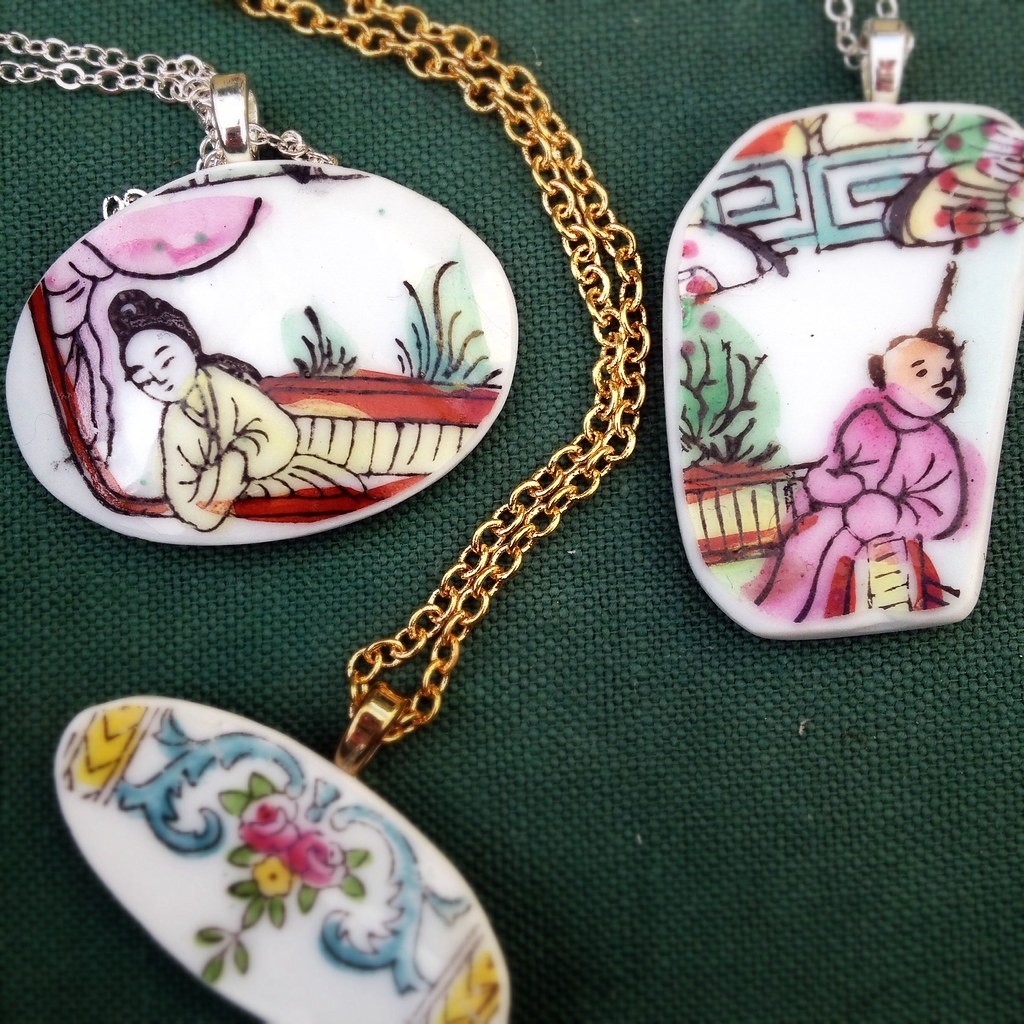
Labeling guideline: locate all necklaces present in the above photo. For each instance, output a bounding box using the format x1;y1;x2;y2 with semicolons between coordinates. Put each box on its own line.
1;29;519;547
53;1;650;1024
661;0;1024;641
